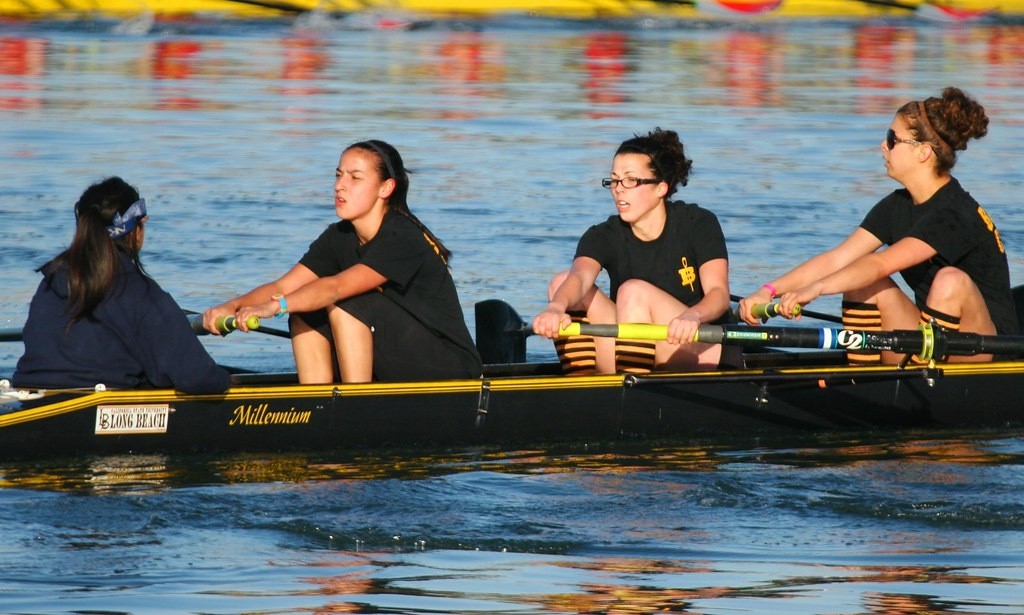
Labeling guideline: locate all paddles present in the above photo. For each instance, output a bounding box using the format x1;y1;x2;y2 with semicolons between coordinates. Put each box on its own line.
928;1;1002;19
557;319;1024;356
0;311;259;343
473;298;802;364
228;0;412;29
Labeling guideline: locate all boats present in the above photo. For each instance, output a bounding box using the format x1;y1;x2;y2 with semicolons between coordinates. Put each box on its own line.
0;288;1024;469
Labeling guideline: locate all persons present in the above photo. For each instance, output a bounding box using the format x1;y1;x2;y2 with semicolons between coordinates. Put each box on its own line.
738;86;1019;366
203;139;484;384
532;127;743;375
8;177;231;396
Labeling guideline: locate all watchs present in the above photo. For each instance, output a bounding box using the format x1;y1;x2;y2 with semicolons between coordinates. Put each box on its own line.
271;293;287;318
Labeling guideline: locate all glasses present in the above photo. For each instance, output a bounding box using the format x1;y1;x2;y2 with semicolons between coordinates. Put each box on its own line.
602;177;662;188
887;128;940;158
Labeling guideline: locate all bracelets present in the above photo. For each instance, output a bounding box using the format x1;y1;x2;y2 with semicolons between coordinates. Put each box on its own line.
763;283;776;298
